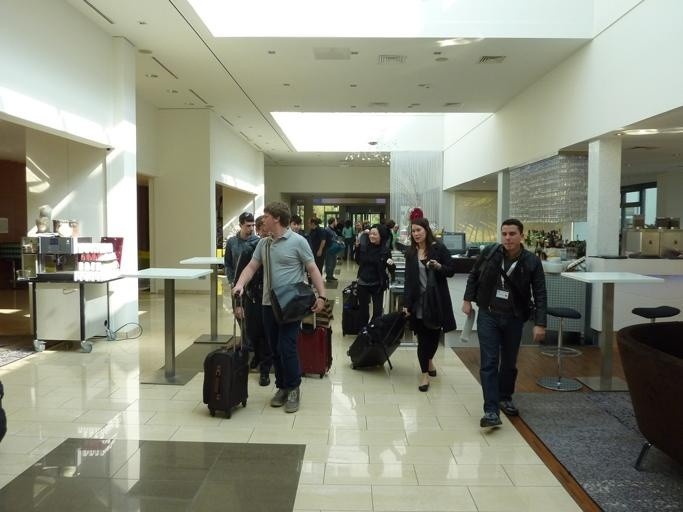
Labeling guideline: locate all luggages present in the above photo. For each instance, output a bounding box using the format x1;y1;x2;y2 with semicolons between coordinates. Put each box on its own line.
298;312;332;378
342;297;370;336
347;311;406;370
202;292;248;419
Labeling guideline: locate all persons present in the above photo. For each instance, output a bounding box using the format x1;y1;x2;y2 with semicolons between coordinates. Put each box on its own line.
463;219;547;427
224;200;457;414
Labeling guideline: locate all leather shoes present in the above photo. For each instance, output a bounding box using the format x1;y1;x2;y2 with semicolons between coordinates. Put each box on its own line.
428;371;436;376
419;384;428;392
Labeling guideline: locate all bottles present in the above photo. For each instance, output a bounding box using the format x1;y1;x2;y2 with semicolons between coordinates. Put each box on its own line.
525;227;565;260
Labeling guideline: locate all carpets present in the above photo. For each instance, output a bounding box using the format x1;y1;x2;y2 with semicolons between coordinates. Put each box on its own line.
511;391;682;512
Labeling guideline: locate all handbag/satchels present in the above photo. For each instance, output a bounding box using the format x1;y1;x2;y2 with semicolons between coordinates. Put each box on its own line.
302;299;334;328
268;282;317;326
342;281;371;306
358;312;405;343
423;268;444;330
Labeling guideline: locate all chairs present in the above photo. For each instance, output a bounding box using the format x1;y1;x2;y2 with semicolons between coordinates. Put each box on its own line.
616;321;681;469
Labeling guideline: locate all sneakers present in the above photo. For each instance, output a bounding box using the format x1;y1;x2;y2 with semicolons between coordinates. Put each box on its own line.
480;412;502;429
271;388;287;406
500;401;518;417
285;387;300;413
260;368;270;386
250;355;259;369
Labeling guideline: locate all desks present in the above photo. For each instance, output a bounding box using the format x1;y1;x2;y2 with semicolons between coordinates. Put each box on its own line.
120;267;214;385
561;271;665;391
179;257;233;343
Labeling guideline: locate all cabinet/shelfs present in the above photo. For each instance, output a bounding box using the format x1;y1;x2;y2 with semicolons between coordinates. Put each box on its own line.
26;273;115;354
641;229;658;259
659;229;682;259
623;229;641;254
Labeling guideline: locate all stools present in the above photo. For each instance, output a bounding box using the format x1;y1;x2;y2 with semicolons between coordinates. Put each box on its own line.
632;306;680;324
535;306;583;393
